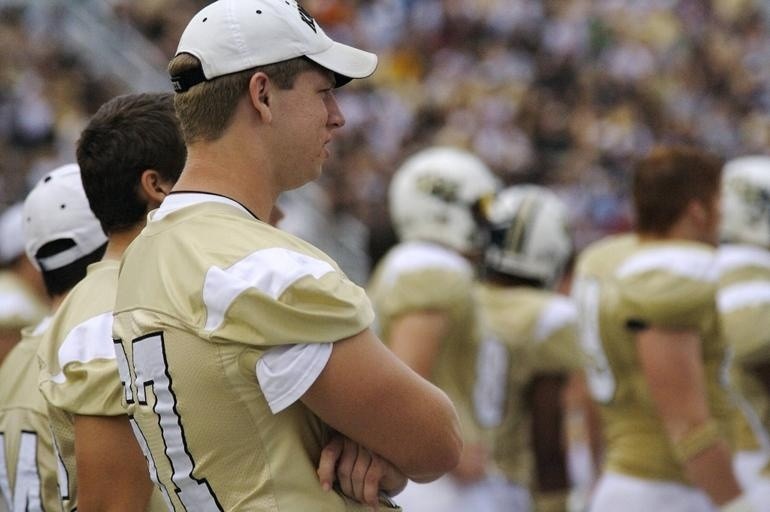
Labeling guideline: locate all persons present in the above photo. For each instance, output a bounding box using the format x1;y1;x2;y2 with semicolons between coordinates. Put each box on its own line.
0;2;767;510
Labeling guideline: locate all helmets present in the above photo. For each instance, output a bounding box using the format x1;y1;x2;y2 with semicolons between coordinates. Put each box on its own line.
387;144;504;259
485;181;576;285
715;152;770;249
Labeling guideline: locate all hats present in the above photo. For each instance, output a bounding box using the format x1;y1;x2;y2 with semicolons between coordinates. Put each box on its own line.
168;0;379;95
0;203;27;266
20;163;108;273
